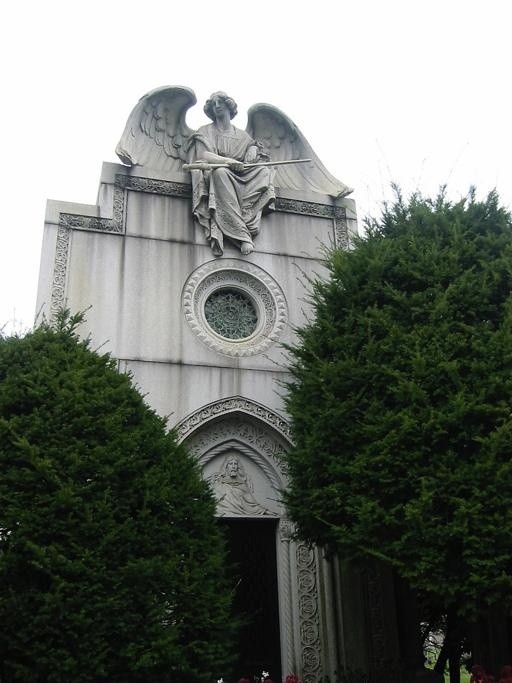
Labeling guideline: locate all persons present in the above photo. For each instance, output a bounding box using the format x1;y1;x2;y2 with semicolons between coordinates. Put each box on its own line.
190;90;276;257
202;455;278;518
